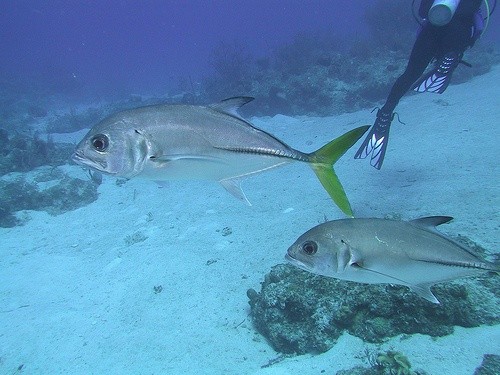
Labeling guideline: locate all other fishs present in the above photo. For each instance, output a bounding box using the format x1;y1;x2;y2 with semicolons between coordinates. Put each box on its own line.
70;96;372;219
285;215;500;304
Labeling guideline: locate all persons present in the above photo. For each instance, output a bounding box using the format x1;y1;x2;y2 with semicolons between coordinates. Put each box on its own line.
353;0;483;170
